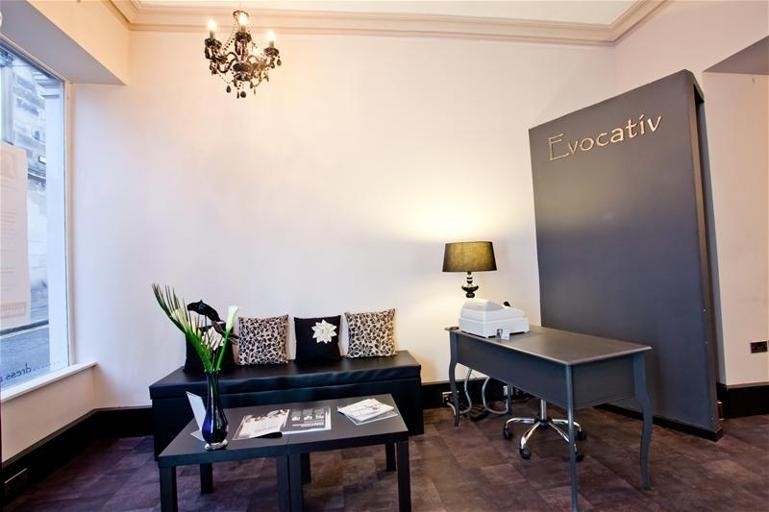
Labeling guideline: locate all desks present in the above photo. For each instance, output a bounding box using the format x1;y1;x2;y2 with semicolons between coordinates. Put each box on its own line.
448;323;652;512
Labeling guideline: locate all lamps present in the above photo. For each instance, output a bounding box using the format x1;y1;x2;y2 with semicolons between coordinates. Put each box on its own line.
442;241;497;298
202;9;281;99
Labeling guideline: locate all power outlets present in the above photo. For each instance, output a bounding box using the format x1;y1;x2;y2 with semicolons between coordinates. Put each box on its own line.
442;391;460;405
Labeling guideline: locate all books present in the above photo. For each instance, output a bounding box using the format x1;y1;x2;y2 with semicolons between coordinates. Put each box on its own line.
232;406;332;441
344;398;398;425
337;398;394;421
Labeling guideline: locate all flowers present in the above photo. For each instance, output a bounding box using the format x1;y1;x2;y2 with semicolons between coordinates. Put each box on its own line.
151;284;240;434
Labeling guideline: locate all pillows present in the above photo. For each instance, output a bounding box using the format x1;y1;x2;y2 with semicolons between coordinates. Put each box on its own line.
345;308;400;358
237;315;289;365
183;322;237;376
293;315;343;365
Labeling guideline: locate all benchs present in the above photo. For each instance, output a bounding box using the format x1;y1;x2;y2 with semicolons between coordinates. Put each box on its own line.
150;351;424;468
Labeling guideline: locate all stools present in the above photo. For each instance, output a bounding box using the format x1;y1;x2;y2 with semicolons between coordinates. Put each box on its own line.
504;398;587;463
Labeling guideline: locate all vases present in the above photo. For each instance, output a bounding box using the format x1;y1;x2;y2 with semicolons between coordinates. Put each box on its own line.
202;370;229;450
